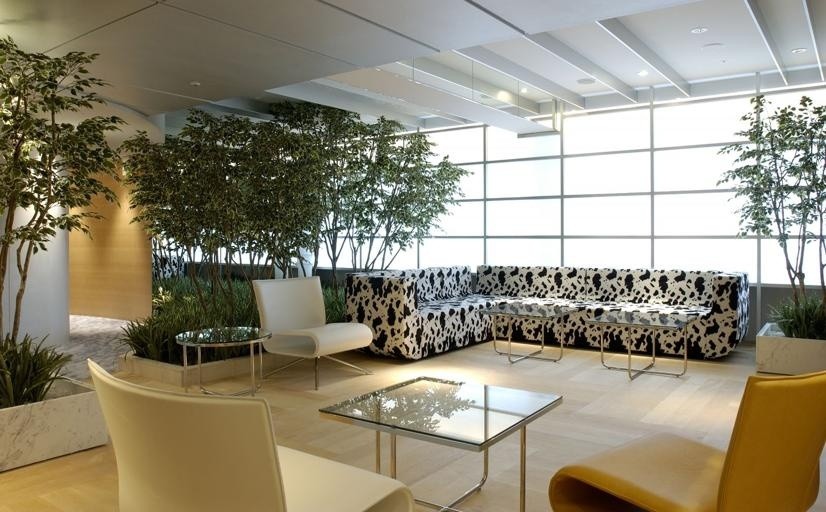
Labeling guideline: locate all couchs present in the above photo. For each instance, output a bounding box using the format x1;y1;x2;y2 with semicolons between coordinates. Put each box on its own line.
346;266;747;361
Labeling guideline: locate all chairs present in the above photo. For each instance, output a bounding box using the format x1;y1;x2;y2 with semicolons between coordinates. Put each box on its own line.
547;370;825;509
86;358;414;512
251;276;374;387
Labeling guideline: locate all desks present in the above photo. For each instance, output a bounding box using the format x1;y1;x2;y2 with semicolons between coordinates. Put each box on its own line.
478;298;580;365
176;326;272;395
583;310;698;380
316;377;562;512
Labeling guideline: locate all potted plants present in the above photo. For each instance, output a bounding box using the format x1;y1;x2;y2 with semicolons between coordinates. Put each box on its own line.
717;88;826;376
0;33;129;474
